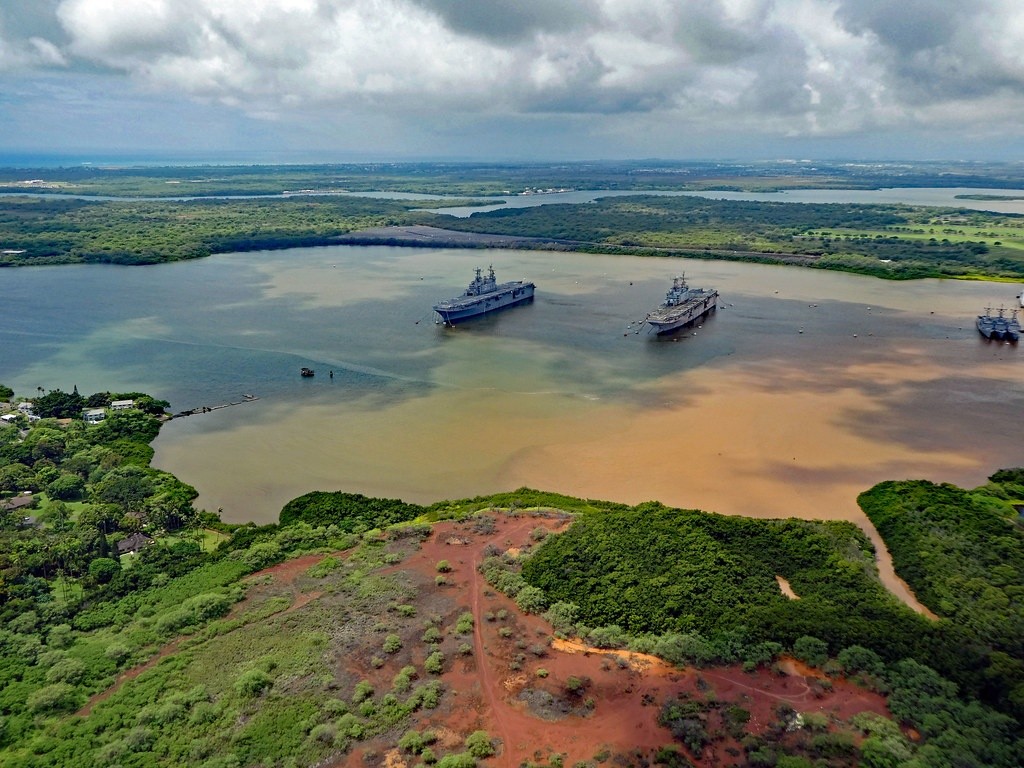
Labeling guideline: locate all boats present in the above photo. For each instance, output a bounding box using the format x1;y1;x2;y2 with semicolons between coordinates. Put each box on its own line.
301;367;315;377
645;272;719;335
432;264;536;325
994;304;1008;338
977;303;994;337
1016;291;1024;308
1009;306;1022;340
